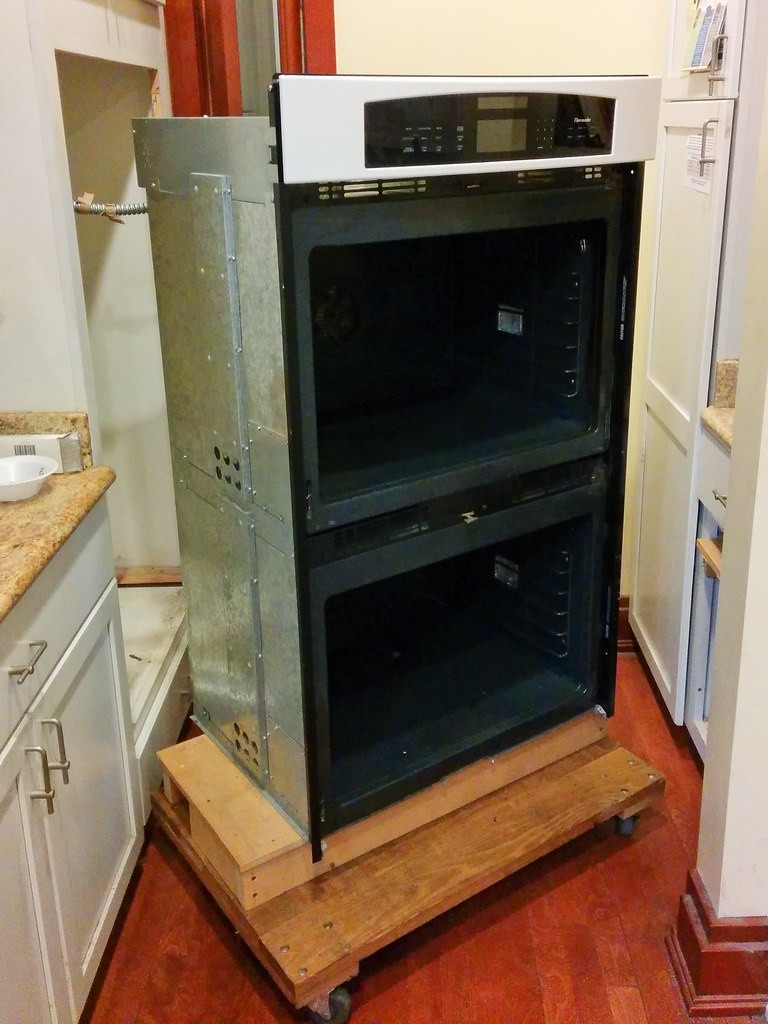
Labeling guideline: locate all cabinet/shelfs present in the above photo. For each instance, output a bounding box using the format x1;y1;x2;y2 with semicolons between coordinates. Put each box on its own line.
0;495;146;1024
627;0;758;727
684;424;731;762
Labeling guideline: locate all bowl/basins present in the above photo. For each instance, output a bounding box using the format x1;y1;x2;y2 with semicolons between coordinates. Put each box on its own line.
0;455;59;502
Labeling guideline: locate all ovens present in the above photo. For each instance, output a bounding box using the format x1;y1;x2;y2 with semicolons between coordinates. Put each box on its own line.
125;72;667;1024
